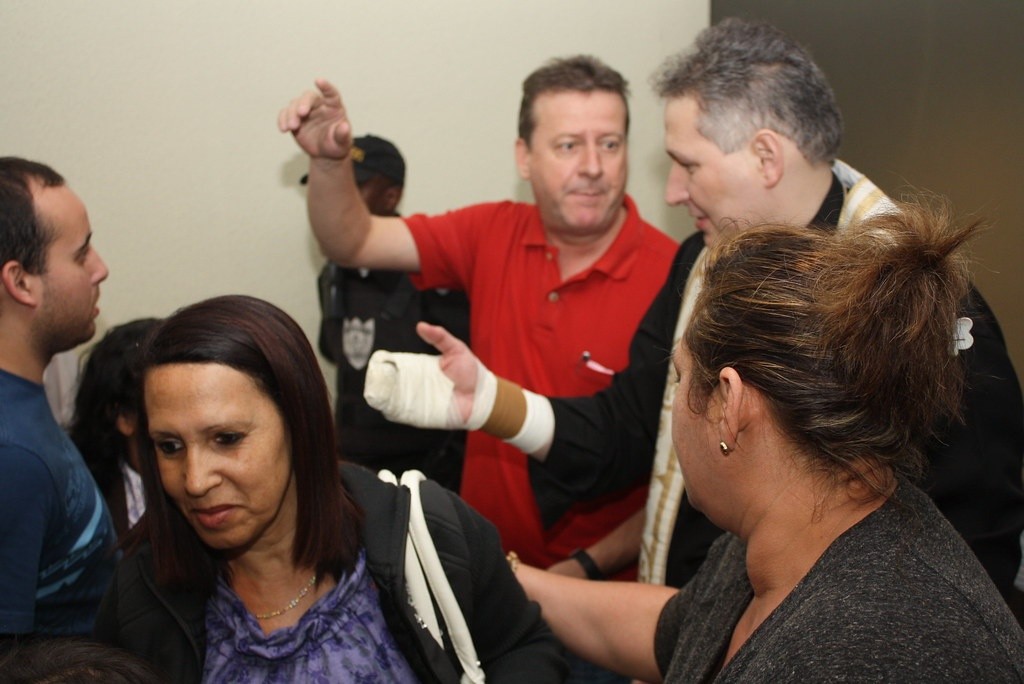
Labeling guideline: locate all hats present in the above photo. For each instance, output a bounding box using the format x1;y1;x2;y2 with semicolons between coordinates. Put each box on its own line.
300;135;405;187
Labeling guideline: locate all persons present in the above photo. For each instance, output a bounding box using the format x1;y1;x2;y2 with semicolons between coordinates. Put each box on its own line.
69;315;168;540
0;155;124;641
502;205;1024;684
0;633;163;684
137;293;566;684
362;16;1024;628
298;135;470;495
276;54;682;582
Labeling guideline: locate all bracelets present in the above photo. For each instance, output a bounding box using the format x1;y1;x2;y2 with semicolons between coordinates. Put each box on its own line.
570;548;605;581
505;551;520;573
